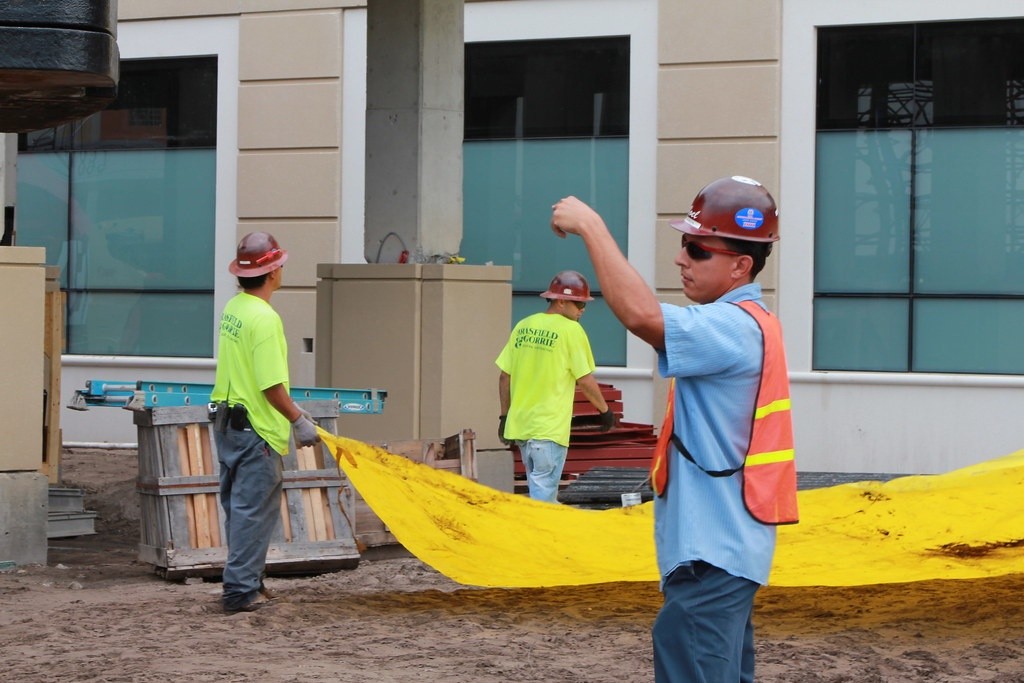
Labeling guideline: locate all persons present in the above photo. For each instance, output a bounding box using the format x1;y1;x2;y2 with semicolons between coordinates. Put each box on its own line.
497;270;614;507
549;176;797;683
207;232;321;613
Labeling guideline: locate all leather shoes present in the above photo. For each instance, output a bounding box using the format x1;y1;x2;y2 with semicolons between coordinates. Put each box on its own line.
223;588;281;614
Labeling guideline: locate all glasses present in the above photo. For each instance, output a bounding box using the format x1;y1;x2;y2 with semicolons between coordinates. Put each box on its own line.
681;233;743;260
571;300;586;310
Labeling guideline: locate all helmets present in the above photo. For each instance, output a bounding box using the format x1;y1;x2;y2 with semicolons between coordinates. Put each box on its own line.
229;231;289;278
669;174;781;243
540;270;595;302
